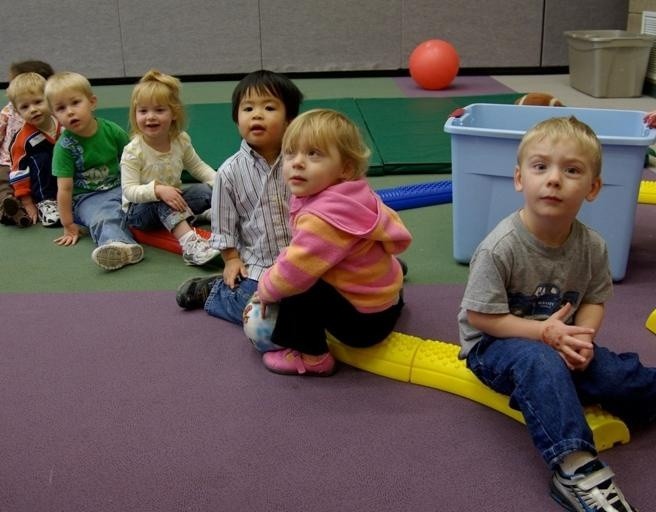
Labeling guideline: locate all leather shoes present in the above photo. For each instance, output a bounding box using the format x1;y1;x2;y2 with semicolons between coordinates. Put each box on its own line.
261;347;336;377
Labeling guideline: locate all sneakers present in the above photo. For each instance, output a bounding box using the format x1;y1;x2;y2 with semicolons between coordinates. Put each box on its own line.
91;241;144;271
548;457;639;512
182;230;221;265
176;274;223;308
2;194;64;228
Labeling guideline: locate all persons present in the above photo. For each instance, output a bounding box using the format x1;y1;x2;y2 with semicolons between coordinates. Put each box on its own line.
4;71;62;227
119;70;222;265
176;69;306;325
0;60;56;227
253;109;414;377
456;116;656;512
43;71;144;270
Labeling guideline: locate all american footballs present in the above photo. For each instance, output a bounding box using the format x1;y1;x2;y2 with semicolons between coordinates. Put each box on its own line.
513;91;565;107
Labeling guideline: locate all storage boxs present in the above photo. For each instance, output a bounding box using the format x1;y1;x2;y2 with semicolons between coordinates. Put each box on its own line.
627;14;656;84
628;0;656;14
564;29;653;98
443;103;656;283
